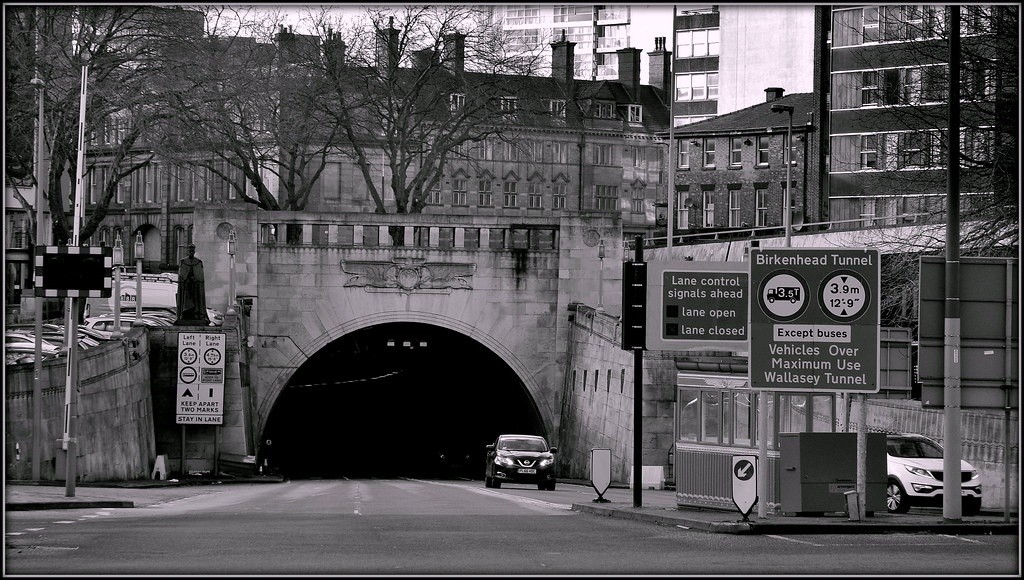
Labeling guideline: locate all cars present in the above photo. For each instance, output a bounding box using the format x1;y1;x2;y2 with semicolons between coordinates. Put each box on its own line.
485;433;558;492
883;433;982;517
5;270;225;359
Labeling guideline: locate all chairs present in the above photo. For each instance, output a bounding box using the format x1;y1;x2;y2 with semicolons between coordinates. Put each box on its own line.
899;444;916;455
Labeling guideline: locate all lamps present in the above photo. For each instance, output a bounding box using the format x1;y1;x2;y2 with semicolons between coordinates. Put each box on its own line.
691;141;700;147
743;138;752;146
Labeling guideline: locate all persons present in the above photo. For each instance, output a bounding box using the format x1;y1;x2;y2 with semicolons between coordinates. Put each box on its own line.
172;244;210;327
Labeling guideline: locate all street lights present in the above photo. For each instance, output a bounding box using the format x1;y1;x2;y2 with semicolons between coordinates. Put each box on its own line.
770;103;795;248
28;76;42;487
